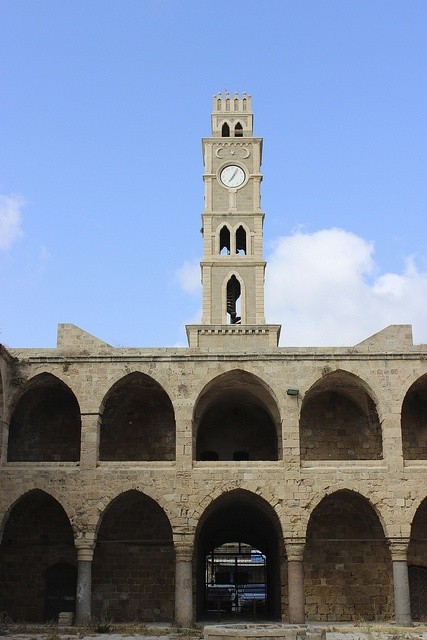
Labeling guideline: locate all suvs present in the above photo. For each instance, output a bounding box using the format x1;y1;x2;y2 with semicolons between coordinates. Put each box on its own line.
206;585;233;612
238;584;267;612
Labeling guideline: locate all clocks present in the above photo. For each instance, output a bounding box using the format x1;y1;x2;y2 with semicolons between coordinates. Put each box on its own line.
220;165;245;189
